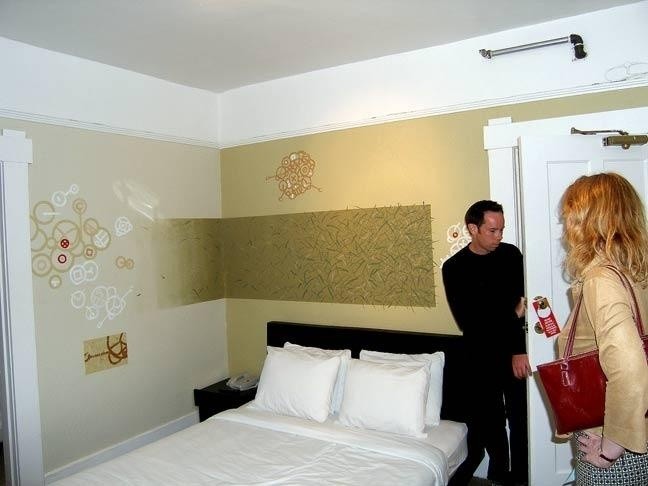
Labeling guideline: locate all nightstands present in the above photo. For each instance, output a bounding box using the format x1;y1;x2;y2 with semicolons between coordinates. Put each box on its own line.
194;378;258;423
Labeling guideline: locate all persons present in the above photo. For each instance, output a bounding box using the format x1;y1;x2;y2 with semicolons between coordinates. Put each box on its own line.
441;201;532;486
536;172;648;486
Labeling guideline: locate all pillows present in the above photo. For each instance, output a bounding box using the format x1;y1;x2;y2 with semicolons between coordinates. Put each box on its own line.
341;356;431;439
283;342;351;422
359;350;445;426
255;345;341;422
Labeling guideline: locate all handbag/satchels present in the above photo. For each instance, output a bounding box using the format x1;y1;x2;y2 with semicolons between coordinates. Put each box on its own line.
535;265;648;432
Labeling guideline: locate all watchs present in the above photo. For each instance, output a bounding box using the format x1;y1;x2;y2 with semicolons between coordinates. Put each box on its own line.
597;447;621;466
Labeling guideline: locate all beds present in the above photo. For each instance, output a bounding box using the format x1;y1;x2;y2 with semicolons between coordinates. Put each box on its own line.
47;321;486;486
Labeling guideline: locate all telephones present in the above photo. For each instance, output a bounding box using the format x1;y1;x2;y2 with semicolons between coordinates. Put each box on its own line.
225;372;260;391
